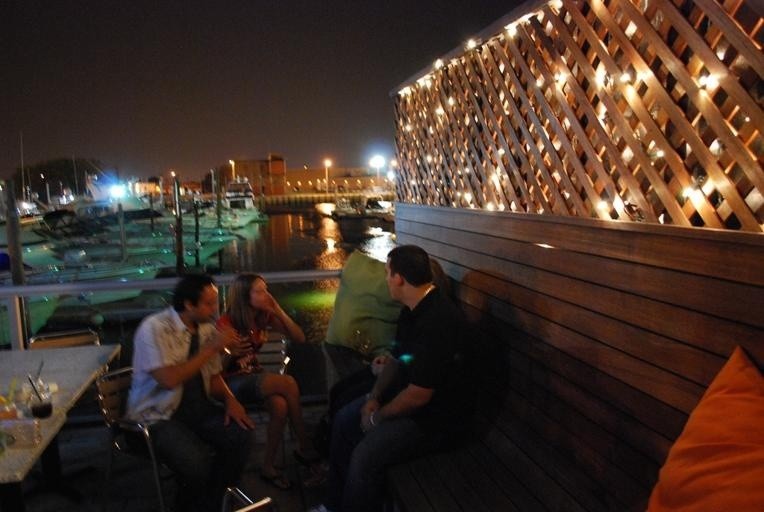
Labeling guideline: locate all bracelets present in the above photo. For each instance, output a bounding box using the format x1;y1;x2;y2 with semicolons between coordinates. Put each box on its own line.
370;411;375;427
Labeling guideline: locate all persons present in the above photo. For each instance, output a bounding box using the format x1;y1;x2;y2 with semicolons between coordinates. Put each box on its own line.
215;272;324;493
311;243;465;512
327;257;447;412
120;271;254;511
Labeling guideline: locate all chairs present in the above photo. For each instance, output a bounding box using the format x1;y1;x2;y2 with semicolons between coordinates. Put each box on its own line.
95;330;290;510
31;331;103;416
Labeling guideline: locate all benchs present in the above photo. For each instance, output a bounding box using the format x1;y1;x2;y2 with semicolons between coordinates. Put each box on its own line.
320;202;763;510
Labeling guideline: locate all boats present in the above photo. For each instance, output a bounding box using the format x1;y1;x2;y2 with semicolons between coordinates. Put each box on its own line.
332;199;395;250
0;197;268;345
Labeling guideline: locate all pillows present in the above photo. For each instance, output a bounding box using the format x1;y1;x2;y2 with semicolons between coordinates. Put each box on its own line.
644;342;764;511
324;248;399;354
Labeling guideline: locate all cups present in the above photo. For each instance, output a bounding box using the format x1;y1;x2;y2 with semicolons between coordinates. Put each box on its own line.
29;392;53;418
213;316;232;334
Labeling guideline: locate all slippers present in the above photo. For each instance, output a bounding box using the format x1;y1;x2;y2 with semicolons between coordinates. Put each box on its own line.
259;470;295;492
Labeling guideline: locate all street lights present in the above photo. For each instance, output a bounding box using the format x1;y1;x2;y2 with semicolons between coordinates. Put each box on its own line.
370;156;383;192
229;159;237;185
323;159;332;192
